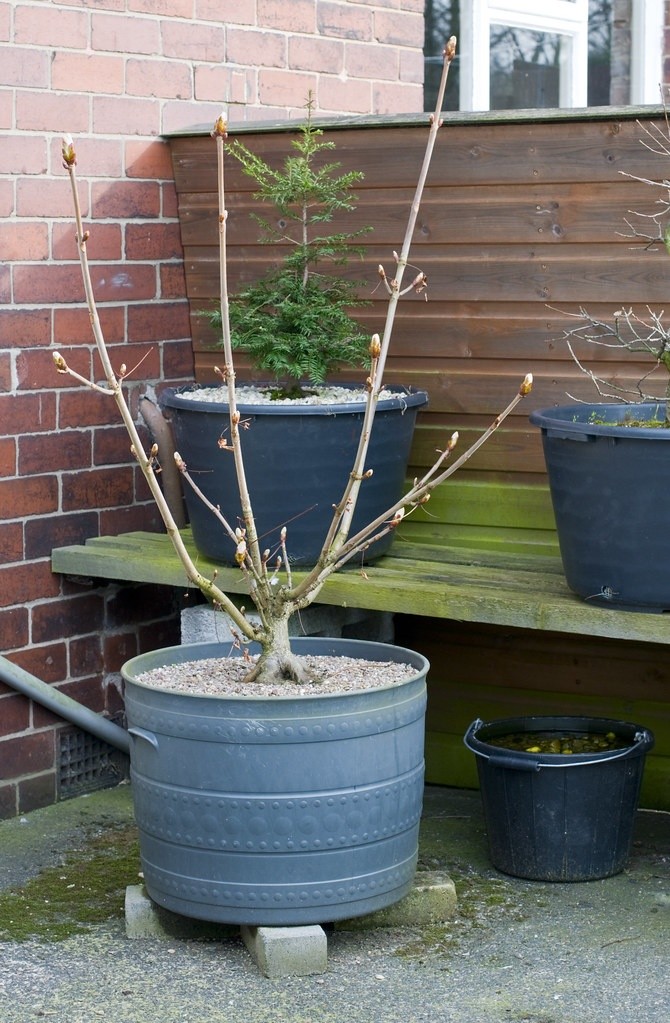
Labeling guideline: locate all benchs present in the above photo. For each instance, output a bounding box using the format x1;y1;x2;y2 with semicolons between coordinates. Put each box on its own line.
50;102;668;650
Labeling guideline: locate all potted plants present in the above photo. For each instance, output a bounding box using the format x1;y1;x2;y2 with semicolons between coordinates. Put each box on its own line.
161;86;432;571
62;33;534;927
531;80;670;613
